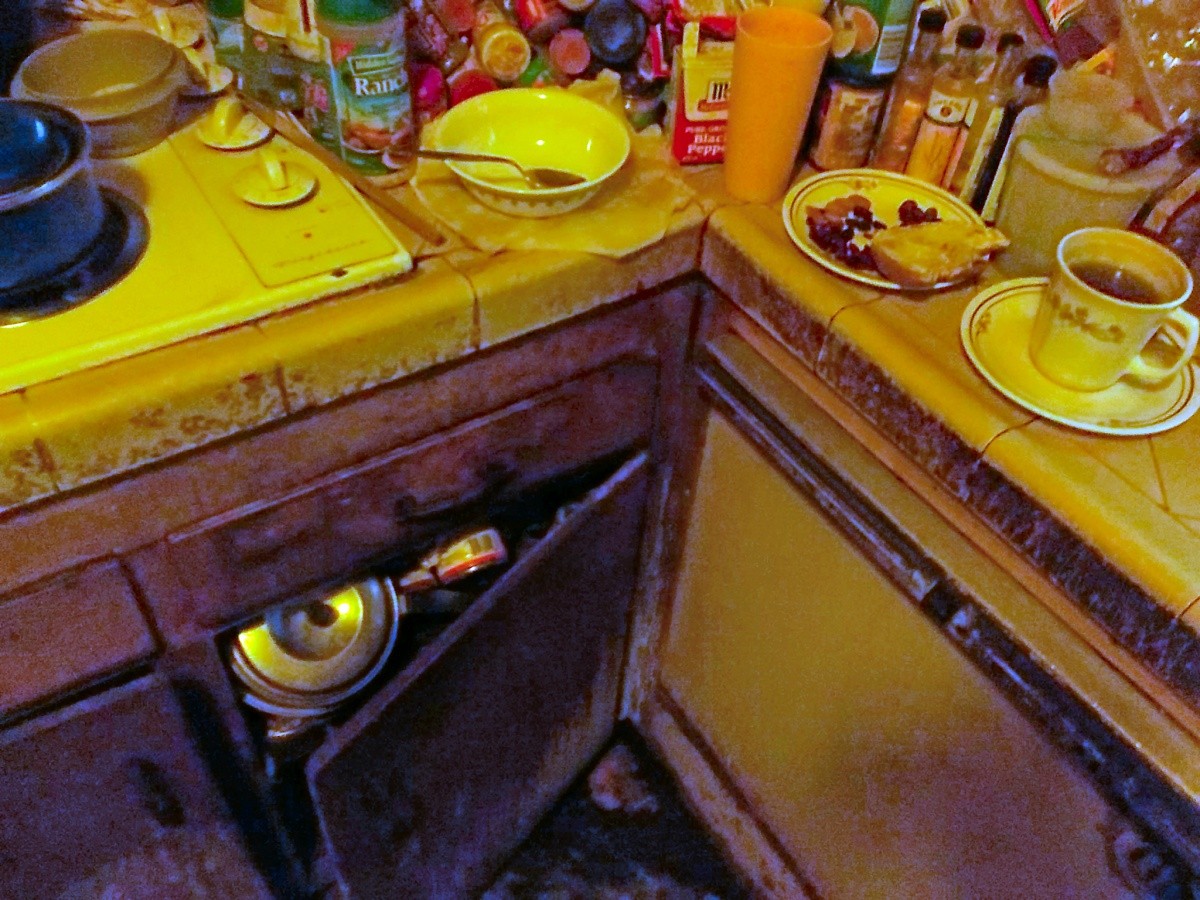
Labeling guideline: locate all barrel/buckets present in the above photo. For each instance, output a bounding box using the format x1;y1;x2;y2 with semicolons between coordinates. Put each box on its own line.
992;113;1180;277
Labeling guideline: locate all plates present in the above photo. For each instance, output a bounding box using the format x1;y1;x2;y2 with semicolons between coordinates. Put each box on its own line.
960;274;1200;438
778;167;990;292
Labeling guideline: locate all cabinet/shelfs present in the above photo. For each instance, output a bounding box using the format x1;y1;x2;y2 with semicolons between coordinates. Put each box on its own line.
0;276;697;899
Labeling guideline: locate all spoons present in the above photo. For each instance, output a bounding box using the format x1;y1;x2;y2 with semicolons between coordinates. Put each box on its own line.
376;146;588;189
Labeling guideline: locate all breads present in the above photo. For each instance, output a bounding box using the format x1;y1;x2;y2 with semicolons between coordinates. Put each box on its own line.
873;220;1012;288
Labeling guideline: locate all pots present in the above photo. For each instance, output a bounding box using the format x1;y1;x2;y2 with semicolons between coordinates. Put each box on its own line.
1;98;102;295
221;577;399;715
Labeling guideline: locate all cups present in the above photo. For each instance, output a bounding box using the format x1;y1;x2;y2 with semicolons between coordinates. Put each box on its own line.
1026;224;1200;390
724;6;834;205
1041;70;1131;136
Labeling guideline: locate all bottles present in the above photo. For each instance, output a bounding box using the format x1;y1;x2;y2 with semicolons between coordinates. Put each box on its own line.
905;22;985;188
965;54;1056;225
864;6;951;177
942;33;1029;211
207;0;682;188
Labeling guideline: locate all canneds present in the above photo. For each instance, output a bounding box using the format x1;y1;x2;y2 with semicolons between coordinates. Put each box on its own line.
806;0;915;172
205;1;657;189
436;530;508;586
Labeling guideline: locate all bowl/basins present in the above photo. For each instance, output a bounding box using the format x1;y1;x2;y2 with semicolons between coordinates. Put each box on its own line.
435;84;630;220
11;31;188;161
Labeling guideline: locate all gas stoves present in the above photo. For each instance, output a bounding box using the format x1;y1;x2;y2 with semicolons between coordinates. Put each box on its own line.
0;94;410;398
